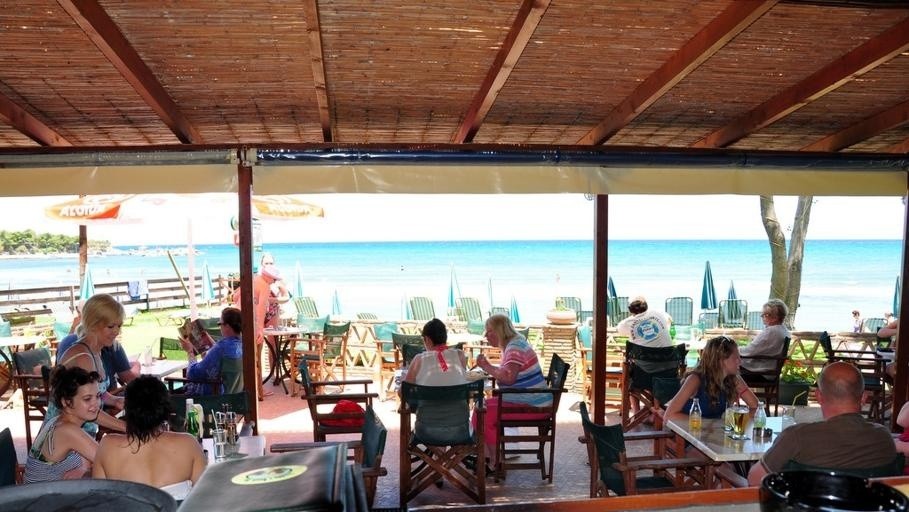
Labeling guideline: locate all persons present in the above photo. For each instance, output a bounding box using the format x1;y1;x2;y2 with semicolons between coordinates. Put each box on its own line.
168;307;243;395
34;293;126;435
748;362;898;487
398;319;467;490
851;310;863;332
55;309;141;385
618;299;674;423
256;253;289;381
738;299;790;382
92;374;207;503
22;365;100;484
469;314;557;477
883;312;897;325
663;336;759;474
233;268;282;347
877;318;900;349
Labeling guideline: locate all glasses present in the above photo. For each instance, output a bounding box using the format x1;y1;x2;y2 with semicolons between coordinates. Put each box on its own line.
217;322;230;325
66;371;99;386
717;336;730;352
262;262;274;265
760;312;769;317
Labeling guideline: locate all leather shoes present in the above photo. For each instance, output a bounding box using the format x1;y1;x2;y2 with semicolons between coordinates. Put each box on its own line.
472;463;502;476
433;479;444;490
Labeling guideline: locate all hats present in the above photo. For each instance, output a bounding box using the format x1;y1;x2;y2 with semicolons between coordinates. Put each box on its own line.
319;399;365;427
261;267;284;281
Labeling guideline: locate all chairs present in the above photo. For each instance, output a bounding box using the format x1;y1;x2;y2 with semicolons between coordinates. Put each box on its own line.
666;296;692;344
13;347;54;455
0;321;14;379
456;297;484;336
622;341;687;433
721;336;792;417
290;292;331;322
390;332;426;403
775;450;904;486
812;330;887;426
576;402;721;499
195;317;224;335
167;390;255;436
515;325;528;344
488;307;509;322
646;374;713;486
568;328;625;412
44;322;72;349
718;300;752;330
286;321;351;398
294;357;378;456
359;312;384;321
282;314;330;389
492;353;573;486
860;315;890;336
395;380;489;506
867;323;896;422
0;428;27;492
555;295;582;320
406;296;434;324
607;296;629;328
265;407;385;512
575;311;595;327
698;311;722;331
461;317;486;339
156;334;198;361
164;355;246;398
365;322;402;401
746;309;769;331
402;341;467;382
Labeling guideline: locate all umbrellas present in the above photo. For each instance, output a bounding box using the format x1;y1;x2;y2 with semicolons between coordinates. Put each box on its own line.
81;264;94;303
47;191;324;319
331;290;341;318
201;262;215;308
447;278;455;312
295;262;303;295
487;278;494;308
404;297;413;321
701;260;718;317
607;277;621;326
726;280;742;325
510;281;519;324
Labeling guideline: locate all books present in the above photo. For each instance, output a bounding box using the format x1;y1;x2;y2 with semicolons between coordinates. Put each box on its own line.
177;318;214;356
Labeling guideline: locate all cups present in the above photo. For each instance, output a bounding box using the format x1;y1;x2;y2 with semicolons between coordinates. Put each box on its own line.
728;405;750;442
724;401;737;434
212;430;227;461
781;406;796;432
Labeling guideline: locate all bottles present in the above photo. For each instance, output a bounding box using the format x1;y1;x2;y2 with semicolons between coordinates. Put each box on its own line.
754;402;767;431
688;398;700;433
184;411;198;443
